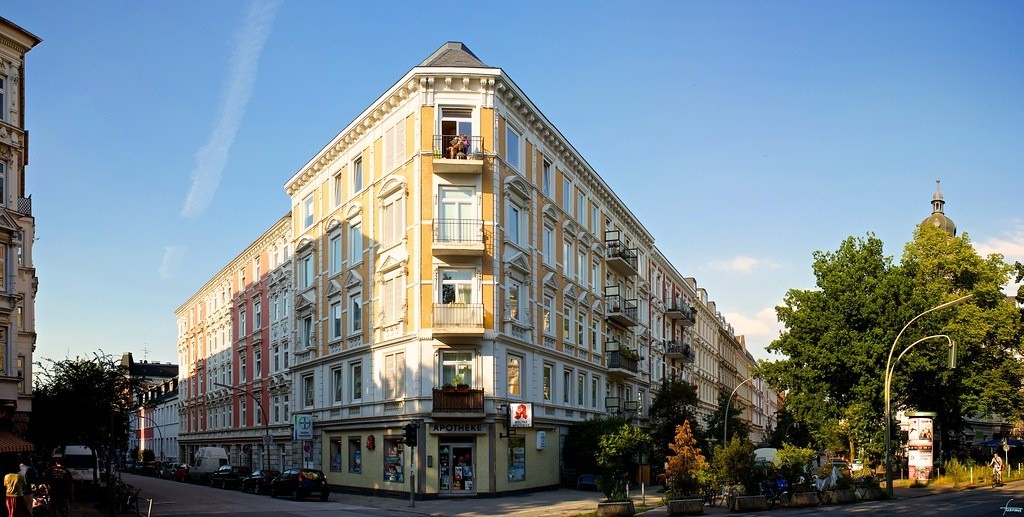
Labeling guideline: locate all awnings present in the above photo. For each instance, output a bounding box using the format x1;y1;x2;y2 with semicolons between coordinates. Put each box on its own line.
0;432;34;453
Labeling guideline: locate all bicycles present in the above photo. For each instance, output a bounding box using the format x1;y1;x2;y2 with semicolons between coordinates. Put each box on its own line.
991;466;1002;489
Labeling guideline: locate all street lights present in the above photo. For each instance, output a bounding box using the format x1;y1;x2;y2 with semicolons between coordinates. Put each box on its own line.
724;373;765;450
884;293;975;498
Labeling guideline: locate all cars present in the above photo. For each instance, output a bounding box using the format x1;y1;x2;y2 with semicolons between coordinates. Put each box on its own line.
122;463;330;504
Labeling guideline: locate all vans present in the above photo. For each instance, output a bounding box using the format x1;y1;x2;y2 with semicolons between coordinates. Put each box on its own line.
62;445;101;482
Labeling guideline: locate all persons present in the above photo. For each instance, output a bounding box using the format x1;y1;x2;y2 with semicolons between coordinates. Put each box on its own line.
988;453;1003;485
4;463;36;517
450;133;470;160
